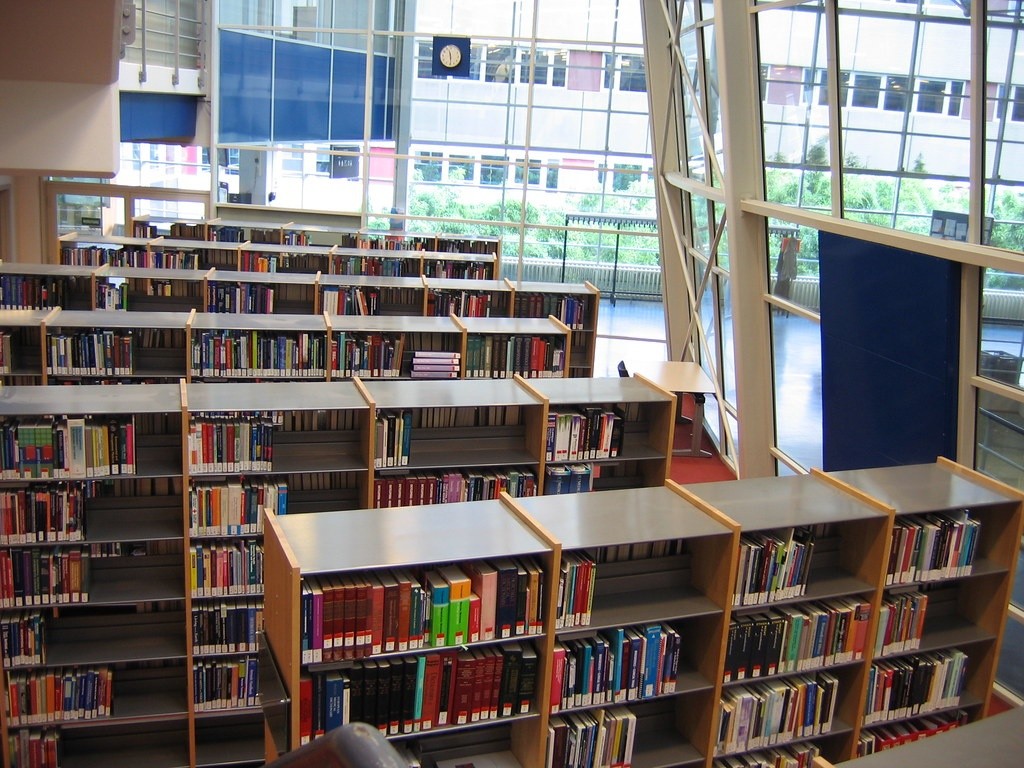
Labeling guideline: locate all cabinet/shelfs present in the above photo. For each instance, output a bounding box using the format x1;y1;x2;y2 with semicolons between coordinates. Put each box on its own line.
0;305;572;385
128;214;503;272
0;371;678;768
258;456;1024;768
55;230;501;280
0;259;601;378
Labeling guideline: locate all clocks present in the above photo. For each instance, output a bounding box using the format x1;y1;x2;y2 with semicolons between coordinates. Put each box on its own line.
432;36;471;77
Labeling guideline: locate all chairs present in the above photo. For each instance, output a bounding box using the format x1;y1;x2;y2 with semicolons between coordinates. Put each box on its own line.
617;360;629;377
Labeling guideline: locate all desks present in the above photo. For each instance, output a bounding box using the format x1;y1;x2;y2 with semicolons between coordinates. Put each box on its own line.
637;360;716;458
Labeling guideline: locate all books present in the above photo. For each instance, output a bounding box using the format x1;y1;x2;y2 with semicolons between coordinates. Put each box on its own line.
0;218;621;768
861;648;969;727
874;585;927;657
300;523;875;768
886;509;980;586
857;710;968;758
